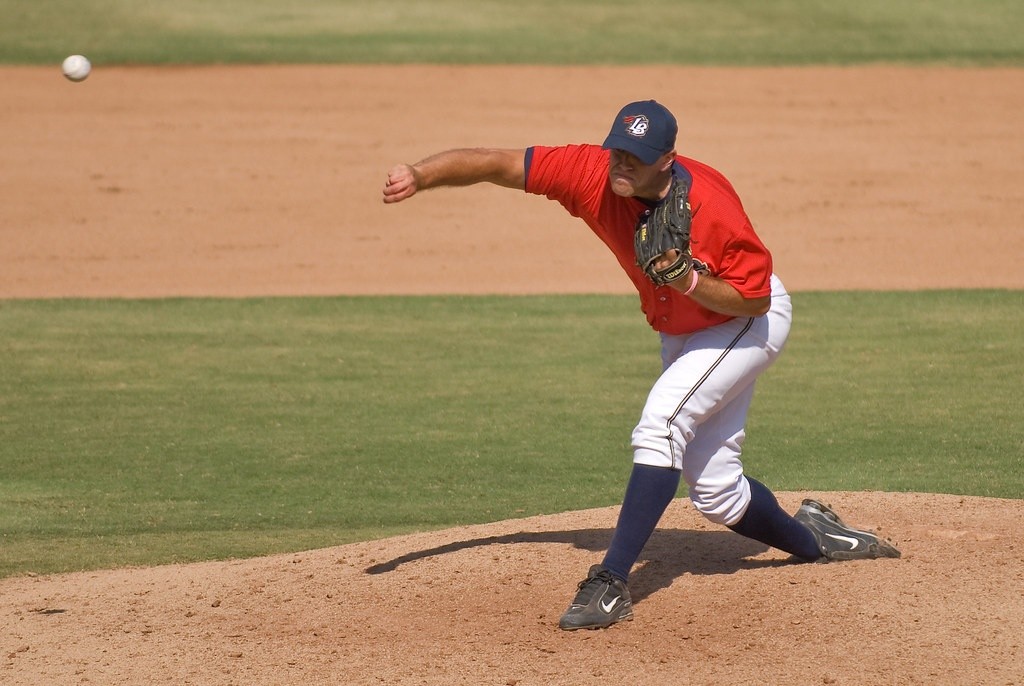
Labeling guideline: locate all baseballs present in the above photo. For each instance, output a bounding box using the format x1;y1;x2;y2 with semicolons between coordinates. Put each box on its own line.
61;53;93;82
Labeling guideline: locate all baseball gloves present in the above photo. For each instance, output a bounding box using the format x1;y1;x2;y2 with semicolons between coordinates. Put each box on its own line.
632;178;693;288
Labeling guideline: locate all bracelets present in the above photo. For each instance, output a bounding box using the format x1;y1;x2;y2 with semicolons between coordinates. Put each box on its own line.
683;270;699;298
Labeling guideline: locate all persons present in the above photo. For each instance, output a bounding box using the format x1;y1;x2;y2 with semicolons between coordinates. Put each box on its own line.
380;99;902;631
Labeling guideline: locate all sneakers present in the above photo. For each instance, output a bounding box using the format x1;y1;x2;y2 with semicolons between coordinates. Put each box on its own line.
558;565;635;630
791;498;901;562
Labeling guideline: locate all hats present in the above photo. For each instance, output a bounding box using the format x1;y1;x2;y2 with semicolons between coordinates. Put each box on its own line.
601;99;679;165
